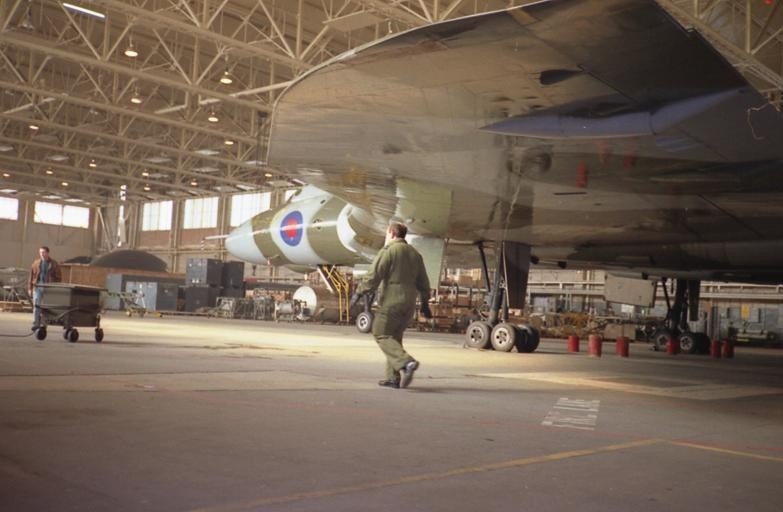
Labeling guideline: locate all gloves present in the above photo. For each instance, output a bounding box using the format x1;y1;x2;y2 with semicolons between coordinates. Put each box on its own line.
420;302;433;319
349;293;360;310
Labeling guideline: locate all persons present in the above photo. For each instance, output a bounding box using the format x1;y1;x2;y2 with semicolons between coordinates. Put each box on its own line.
27;246;62;331
347;222;434;389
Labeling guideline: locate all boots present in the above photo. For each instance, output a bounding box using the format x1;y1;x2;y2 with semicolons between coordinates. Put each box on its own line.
31;325;38;330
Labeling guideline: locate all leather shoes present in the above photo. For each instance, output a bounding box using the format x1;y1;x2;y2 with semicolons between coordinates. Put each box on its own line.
399;362;419;389
378;381;398;388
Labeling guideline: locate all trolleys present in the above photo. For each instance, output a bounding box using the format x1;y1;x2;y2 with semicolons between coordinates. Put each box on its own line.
107;292;147;318
31;283;108;342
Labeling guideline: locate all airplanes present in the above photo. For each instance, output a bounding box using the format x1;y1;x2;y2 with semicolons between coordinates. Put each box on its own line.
226;0;783;354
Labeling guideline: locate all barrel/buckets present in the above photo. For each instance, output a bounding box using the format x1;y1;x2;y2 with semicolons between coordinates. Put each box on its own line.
589;334;602;357
722;337;735;359
567;334;580;353
666;338;680;354
616;335;629;357
710;336;721;356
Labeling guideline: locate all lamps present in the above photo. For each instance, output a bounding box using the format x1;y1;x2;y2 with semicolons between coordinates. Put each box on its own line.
124;40;139;56
219;65;233;84
130;84;143;103
62;2;105;19
21;13;34;29
1;120;274;192
208;106;219;123
